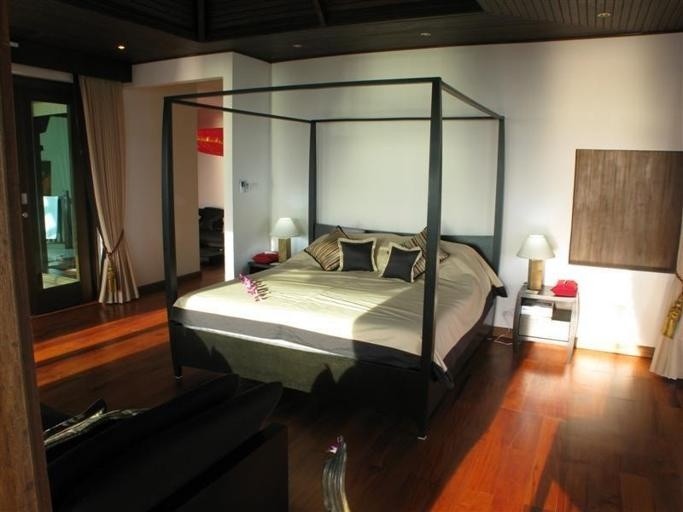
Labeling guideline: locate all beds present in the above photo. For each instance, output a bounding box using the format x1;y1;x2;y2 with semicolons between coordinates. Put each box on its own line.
170;223;493;442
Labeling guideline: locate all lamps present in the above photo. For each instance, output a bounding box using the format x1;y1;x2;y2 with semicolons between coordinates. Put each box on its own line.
269;217;299;263
518;233;554;289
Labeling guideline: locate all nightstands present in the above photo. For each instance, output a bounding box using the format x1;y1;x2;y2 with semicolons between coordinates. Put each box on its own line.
248;261;276;275
513;282;580;360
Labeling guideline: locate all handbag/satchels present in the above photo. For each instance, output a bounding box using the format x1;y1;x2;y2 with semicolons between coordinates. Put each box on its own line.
550;279;578;297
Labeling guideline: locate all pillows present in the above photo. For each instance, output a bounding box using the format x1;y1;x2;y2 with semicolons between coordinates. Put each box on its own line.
304;226;448;283
